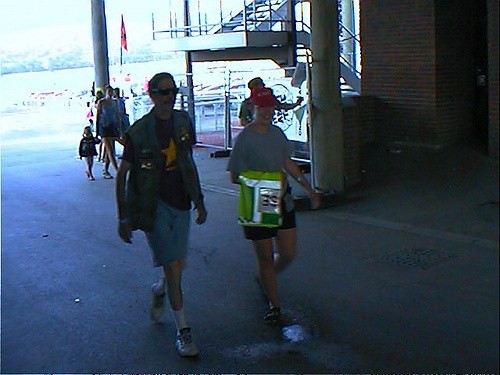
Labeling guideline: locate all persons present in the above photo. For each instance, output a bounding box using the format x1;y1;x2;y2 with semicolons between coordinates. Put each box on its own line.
226;88;322;326
239;77;304;127
116;72;207;357
77;85;131;181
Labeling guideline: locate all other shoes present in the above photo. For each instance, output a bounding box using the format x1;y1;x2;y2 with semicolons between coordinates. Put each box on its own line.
102;171;112;179
264;306;281;323
85;170;95;180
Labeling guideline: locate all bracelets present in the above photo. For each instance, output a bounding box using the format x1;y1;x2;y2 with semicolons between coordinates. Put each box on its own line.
293;173;305;182
117;215;129;224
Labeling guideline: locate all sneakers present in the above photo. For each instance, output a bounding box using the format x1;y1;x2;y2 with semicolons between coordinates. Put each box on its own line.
151;284;164;322
176;329;200;357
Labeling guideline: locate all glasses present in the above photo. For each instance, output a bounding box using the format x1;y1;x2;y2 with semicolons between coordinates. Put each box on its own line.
151;88;180;95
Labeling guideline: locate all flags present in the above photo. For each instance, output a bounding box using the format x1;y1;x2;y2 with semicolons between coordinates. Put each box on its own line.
121;14;129;52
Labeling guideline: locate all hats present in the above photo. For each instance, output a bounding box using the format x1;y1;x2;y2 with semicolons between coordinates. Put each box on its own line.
251;88;279;106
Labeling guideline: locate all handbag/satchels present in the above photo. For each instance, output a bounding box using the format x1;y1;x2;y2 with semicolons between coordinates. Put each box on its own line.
125;161;164;231
